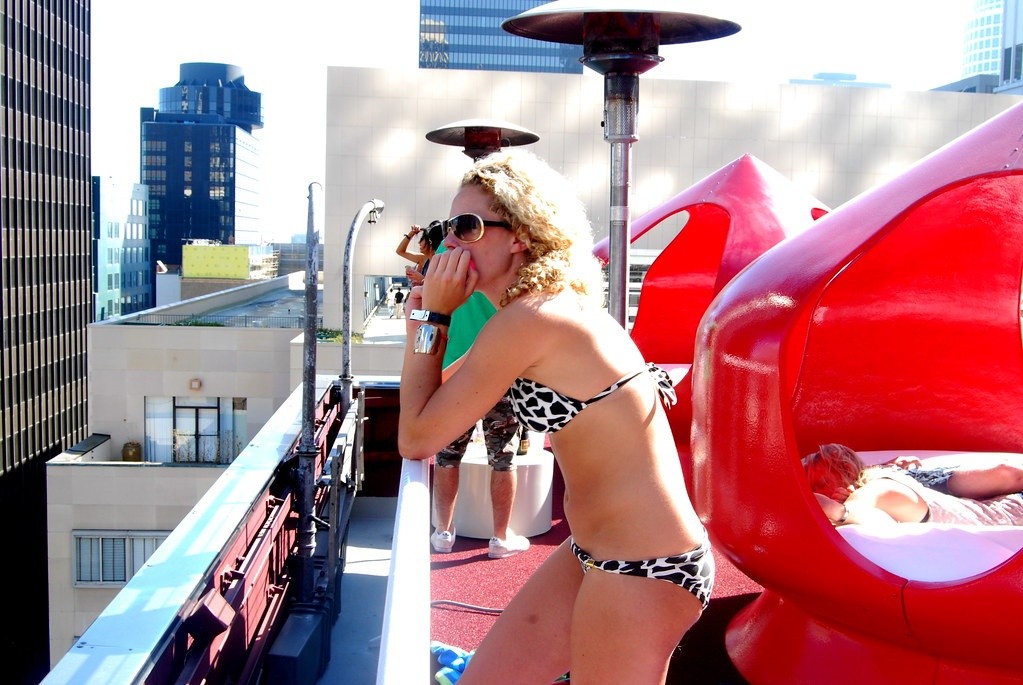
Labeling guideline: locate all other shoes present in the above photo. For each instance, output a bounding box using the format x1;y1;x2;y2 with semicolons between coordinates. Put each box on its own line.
390;315;393;318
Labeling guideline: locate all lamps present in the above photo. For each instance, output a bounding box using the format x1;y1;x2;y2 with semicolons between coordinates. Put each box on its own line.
191;379;201;389
374;283;378;288
365;292;369;297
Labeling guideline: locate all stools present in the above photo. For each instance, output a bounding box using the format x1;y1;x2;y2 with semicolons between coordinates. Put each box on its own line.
434;443;553;539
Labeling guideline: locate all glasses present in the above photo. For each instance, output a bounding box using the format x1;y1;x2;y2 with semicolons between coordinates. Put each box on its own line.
441;213;509;244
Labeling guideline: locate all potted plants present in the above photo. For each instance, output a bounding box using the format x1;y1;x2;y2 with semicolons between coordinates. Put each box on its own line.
120;440;141;461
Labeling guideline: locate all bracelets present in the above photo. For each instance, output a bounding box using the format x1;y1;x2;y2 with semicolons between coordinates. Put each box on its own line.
840;504;849;522
409;309;452;327
420;279;424;284
403;234;411;240
412;323;448;355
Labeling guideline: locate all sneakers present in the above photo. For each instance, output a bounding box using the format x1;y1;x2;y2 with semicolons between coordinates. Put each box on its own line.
488;528;530;558
430;526;456;553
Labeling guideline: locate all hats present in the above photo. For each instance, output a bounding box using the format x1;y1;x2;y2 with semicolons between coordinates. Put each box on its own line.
419;220;441;234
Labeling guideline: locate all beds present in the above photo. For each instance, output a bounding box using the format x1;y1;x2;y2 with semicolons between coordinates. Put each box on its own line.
592;102;1023;685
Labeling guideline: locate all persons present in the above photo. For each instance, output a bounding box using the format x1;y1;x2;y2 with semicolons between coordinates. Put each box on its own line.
396;152;715;685
804;443;1023;528
386;287;411;319
398;220;530;558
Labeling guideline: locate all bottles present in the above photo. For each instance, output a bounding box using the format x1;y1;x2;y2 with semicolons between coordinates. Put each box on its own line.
516;431;529;455
